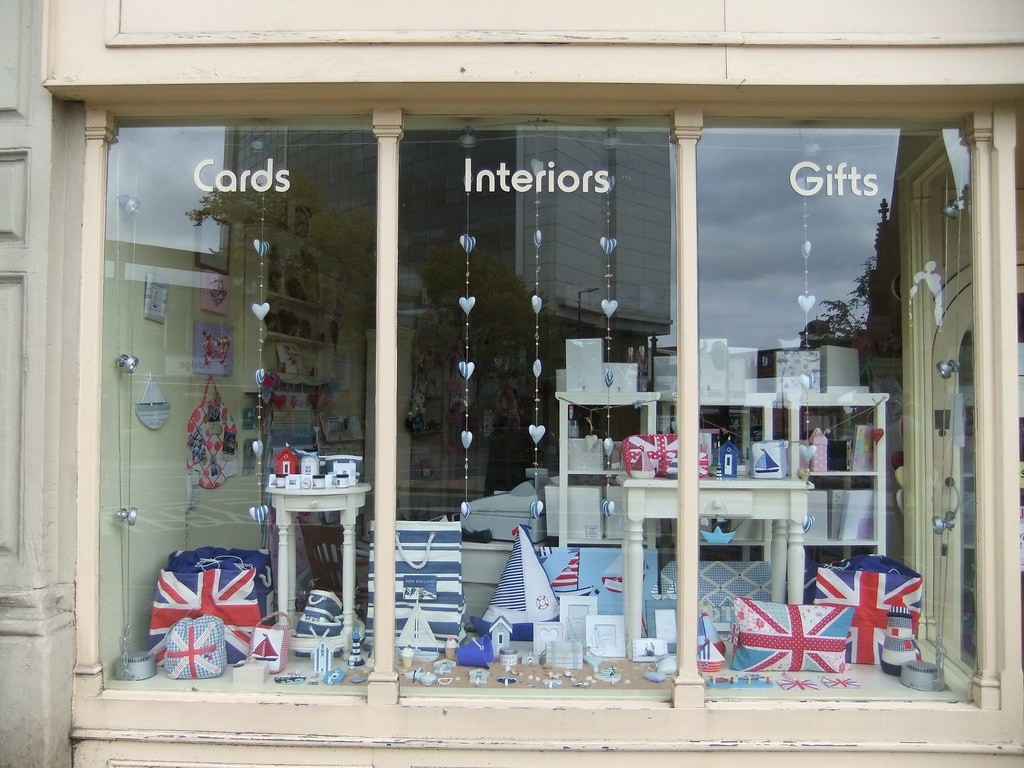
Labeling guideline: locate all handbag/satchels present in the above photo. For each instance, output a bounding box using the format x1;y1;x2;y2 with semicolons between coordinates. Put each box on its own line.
249;611;291;672
364;519;466;655
145;554;261;668
815;554;923;664
165;615;227;679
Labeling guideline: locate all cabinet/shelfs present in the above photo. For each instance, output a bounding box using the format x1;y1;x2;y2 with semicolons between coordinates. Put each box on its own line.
243;222;350;396
363;327;449;539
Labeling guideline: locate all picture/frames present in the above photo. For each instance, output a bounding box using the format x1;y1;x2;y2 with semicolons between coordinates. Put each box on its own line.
144;273;170;324
194;211;232;276
644;599;677;653
559;595;598;648
533;622;564;656
585;614;627;657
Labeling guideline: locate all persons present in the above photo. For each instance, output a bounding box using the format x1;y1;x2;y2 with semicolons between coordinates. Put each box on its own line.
484;386;542;495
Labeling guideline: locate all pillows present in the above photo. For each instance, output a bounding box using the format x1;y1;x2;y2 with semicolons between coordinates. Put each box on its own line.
149;567;262;664
728;596;857;674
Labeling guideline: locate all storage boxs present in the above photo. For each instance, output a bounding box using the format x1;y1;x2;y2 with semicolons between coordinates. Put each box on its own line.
544;483;605;540
603;485;661;541
603;362;643;393
565;337;605;392
652;337;861;392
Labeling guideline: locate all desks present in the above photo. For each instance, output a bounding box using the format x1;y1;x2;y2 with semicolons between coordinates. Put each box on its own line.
656;390;778;562
781;390;890;555
554;390;661;548
264;482;373;662
615;474;816;660
